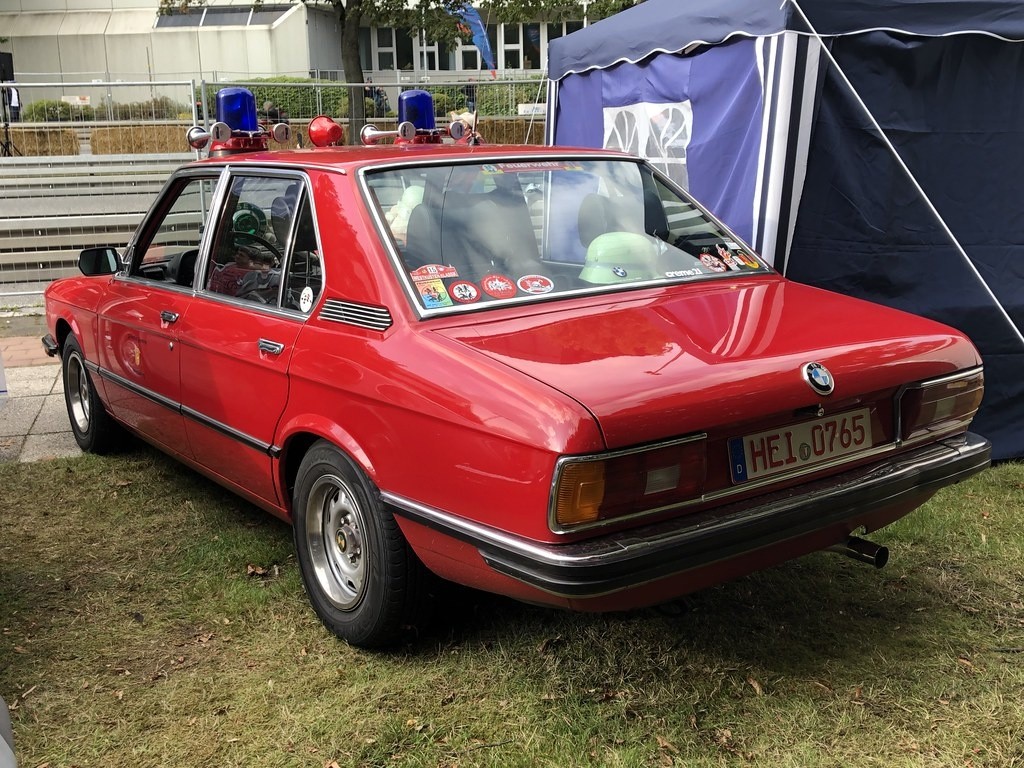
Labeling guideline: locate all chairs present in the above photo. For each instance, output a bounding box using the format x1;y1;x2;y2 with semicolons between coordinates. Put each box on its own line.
406;165;540;280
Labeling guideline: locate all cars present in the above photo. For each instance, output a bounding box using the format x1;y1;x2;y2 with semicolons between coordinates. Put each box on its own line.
41;86;993;651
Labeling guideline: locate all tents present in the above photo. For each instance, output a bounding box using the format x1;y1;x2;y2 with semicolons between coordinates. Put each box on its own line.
543;0;1024;462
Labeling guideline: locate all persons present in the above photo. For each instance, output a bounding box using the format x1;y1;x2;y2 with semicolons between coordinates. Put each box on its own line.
365;76;391;119
3;81;24;123
216;245;260;297
256;101;290;125
461;77;479;114
442;112;486;194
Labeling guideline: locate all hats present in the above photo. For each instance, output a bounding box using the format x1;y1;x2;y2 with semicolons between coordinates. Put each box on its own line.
579;231;667;284
456;112;479;126
239;246;254;258
